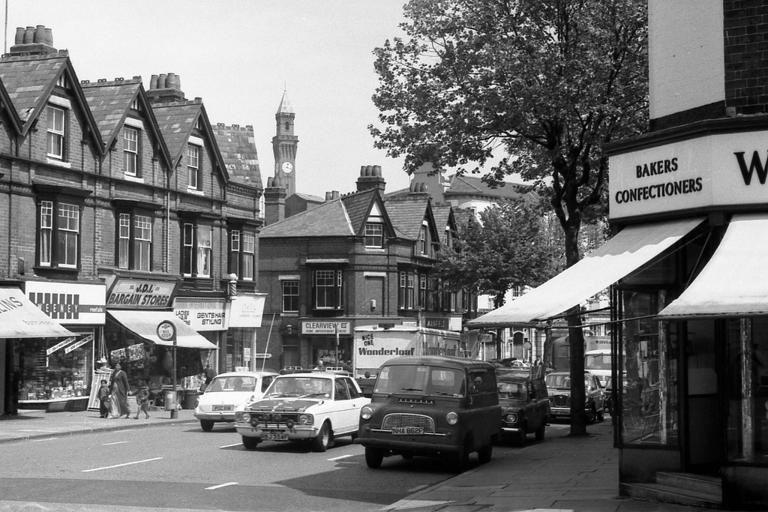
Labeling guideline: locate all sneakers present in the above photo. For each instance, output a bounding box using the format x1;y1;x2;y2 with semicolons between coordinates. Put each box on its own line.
99;412;152;419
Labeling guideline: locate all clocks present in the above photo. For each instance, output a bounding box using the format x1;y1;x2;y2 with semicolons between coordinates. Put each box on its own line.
282;161;293;174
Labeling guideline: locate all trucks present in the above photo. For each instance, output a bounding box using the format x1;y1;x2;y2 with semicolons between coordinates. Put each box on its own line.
349;324;482;401
540;330;626;393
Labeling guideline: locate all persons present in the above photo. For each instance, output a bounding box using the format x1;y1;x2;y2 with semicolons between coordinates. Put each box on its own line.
110;363;130;418
95;379;110;418
128;379;150;419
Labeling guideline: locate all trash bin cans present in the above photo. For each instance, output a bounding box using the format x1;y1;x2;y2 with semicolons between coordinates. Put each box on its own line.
165;389;177;411
181;389;197;409
